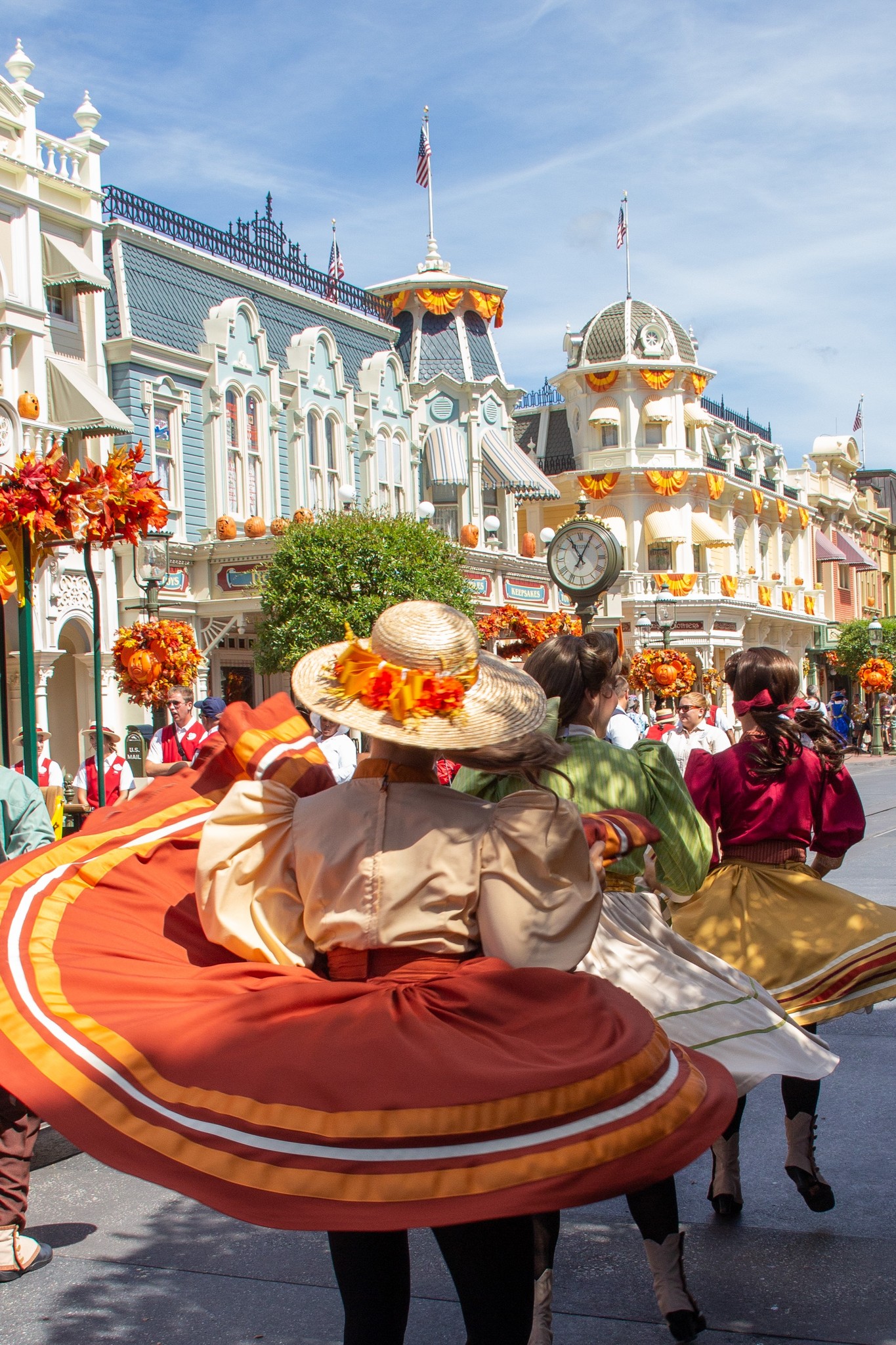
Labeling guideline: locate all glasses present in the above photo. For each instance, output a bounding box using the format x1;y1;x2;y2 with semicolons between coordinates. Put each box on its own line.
675;705;702;714
165;701;189;708
199;714;210;719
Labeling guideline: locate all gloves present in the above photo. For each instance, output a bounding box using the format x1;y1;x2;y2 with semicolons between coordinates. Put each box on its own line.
849;719;854;729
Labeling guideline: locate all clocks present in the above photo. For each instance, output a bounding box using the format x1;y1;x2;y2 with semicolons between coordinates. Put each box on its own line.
547;489;622;635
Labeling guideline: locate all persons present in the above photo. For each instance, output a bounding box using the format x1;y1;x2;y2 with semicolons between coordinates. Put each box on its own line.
639;709;679;742
9;722;65;796
602;676;640;750
71;720;136;814
191;697;228;769
658;692;732;780
0;602;740;1345
647;699;657;727
664;645;896;1227
674;705;737;746
796;684;828;748
144;684;208;778
626;699;646;733
849;693;871;753
447;630;841;1345
0;765;59;864
826;686;855;749
309;711;357;784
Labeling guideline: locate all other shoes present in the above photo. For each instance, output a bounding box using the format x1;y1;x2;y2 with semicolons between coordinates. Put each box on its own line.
0;1242;53;1282
857;748;867;753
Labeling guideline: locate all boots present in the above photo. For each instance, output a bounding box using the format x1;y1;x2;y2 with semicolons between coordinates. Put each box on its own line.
529;1268;554;1345
706;1130;744;1214
642;1230;707;1343
785;1111;835;1213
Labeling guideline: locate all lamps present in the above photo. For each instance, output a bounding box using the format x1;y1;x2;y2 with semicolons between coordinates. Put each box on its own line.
484;515;500;542
540;527;555;552
418;502;435;525
339;484;356;516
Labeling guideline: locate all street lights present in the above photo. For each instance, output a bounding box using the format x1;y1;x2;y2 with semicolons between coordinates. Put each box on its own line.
649;581;684;716
124;532;181;736
868;616;888;755
635;611;653;728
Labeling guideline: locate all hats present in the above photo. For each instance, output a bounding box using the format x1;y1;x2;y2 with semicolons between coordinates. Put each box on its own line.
653;708;678;725
291;600;547;749
831;692;845;699
81;721;121;743
309;711;350;737
193;696;227;720
12;723;52;746
